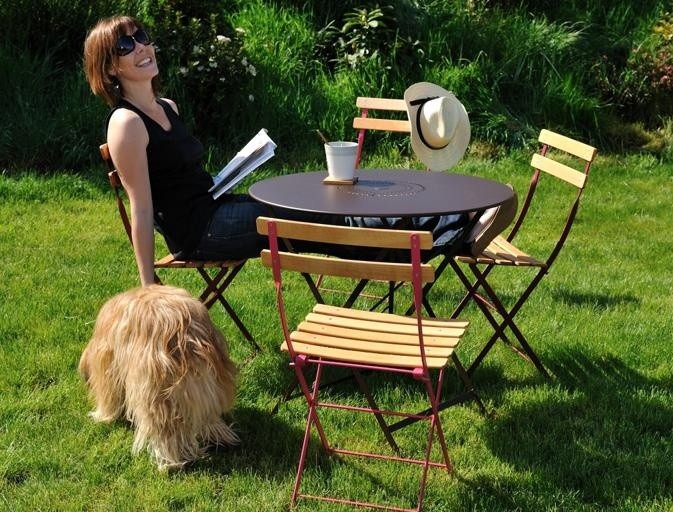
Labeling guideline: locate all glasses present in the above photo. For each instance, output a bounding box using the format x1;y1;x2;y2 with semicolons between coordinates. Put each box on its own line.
110;28;152;57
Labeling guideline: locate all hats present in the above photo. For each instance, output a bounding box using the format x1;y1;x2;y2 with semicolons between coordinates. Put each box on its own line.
403;81;472;173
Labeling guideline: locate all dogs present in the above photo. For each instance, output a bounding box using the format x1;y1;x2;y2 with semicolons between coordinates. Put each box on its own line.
77;282;244;475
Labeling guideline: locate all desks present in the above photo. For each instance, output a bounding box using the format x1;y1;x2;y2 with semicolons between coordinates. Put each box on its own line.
249;169;516;457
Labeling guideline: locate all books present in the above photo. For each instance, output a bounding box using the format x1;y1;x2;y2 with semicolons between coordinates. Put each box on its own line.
207;128;278;202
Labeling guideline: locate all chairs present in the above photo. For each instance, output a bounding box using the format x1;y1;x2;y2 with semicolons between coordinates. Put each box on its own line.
256;217;470;510
99;141;260;352
441;129;598;390
312;96;411;302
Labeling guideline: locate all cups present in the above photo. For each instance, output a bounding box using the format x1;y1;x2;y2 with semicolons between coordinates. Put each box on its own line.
322;141;359;181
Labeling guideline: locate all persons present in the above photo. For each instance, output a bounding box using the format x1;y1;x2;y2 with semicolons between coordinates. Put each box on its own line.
80;15;518;294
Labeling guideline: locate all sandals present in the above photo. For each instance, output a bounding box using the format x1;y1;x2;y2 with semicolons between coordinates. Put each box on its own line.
468;182;519;259
